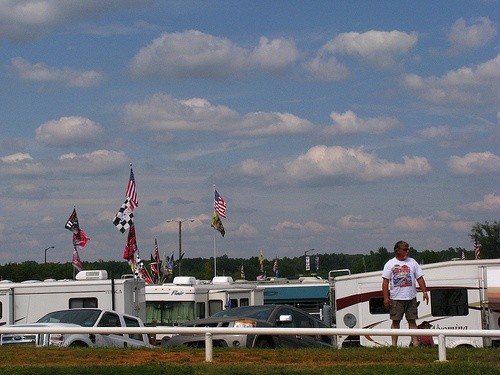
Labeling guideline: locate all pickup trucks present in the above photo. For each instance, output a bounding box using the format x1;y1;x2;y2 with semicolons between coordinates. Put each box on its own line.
0;307;163;350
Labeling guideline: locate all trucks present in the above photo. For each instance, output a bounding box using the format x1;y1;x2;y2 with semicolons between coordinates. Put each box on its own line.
145;258;500;349
0;269;147;325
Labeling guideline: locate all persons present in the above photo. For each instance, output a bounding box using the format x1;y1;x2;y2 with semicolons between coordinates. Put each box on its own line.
382;241;429;348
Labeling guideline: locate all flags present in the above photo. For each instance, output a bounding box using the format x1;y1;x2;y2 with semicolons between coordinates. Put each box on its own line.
211;212;225;237
474;239;480;259
259;254;264;272
64;209;89;271
112;168;151;284
214;190;226;218
273;259;279;278
151;241;174;276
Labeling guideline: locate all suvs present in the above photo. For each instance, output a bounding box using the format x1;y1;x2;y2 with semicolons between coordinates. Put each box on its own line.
168;304;337;350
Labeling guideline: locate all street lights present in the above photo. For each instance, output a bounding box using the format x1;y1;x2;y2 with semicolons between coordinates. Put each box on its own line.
304;249;314;274
44;246;55;264
166;219;194;277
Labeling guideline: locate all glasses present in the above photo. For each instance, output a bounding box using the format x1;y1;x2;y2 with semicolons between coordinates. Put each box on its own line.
398;248;409;252
